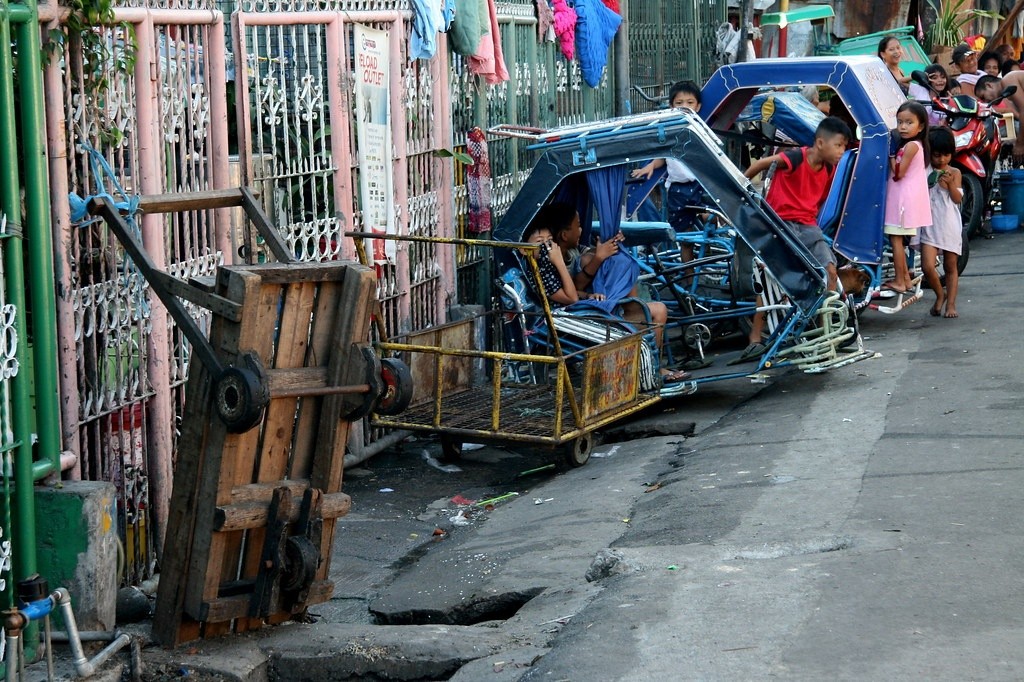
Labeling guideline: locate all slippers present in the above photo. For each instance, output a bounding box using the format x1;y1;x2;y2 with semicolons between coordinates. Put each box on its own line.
906;288;913;292
884;282;907;295
667;357;713;369
661;367;691;382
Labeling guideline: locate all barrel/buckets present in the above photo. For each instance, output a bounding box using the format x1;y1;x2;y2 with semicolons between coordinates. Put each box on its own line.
999;169;1024;224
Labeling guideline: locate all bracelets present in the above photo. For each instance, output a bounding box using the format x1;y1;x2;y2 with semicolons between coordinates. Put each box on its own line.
582;265;595;279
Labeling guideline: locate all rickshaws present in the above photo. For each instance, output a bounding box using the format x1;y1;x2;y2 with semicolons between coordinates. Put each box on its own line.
473;55;959;398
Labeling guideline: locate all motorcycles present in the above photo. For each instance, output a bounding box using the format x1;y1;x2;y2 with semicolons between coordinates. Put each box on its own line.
909;70;1017;243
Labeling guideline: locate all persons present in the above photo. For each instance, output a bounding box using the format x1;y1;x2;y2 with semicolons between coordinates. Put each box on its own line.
773;0;1024;168
519;201;692;392
919;125;963;318
743;116;852;353
884;102;933;294
630;81;721;297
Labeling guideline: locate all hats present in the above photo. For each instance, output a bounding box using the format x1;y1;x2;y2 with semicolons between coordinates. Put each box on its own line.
948;44;977;65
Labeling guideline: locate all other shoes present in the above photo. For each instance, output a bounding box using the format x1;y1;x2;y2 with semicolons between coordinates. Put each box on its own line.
741;342;765;359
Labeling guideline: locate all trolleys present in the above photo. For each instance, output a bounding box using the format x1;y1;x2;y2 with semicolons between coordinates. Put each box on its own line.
85;186;414;650
343;230;667;467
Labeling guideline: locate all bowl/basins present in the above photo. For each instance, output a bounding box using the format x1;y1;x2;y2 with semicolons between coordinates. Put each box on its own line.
991;216;1019;230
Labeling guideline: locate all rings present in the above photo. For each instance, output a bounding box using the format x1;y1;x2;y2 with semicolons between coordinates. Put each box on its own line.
547;247;551;250
